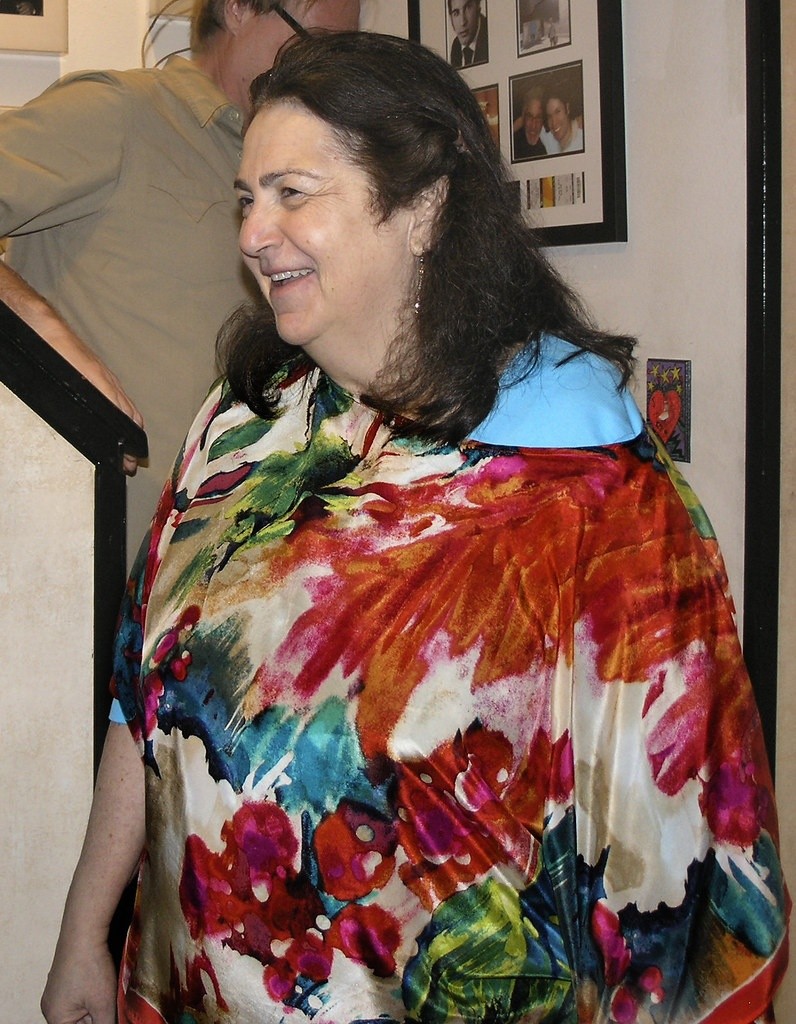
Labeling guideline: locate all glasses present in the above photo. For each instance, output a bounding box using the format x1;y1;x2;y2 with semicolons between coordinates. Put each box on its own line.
527;113;545;121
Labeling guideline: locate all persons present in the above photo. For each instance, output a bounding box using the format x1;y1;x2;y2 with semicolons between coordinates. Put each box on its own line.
42;29;790;1023
1;0;362;582
548;17;558;47
447;0;487;68
513;86;582;159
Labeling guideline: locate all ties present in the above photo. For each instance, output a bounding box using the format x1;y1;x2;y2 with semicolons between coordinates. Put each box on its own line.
463;46;473;65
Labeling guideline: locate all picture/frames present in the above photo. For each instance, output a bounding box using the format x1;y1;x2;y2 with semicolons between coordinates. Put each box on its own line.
408;0;628;248
